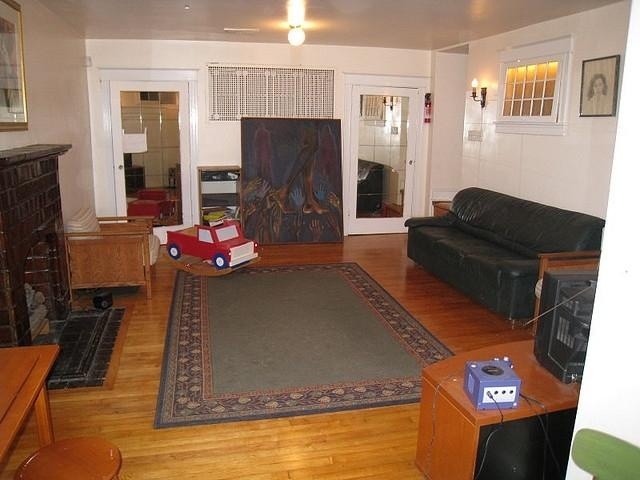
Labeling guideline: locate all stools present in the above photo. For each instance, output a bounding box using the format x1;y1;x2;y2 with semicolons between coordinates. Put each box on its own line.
15;436;122;479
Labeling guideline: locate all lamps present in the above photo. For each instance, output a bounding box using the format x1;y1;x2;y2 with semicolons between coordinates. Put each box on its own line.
382;96;399;109
469;79;487;109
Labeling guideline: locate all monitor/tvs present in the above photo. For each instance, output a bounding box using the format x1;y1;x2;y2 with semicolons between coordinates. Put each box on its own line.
533;271;598;384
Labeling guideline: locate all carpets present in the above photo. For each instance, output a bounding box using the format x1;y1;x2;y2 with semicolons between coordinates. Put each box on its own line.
152;261;462;432
35;303;135;393
126;199;168;217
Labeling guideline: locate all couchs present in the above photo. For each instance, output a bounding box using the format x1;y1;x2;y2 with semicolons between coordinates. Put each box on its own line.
64;203;161;300
357;163;384;208
403;187;605;321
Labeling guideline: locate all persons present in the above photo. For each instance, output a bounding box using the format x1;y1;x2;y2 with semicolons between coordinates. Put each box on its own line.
583;73;612;114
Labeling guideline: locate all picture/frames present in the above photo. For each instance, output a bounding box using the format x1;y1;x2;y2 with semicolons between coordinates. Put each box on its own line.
579;55;620;117
0;0;29;132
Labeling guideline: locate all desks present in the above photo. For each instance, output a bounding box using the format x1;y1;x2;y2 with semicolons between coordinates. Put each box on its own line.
413;339;580;480
1;345;65;480
431;200;452;217
